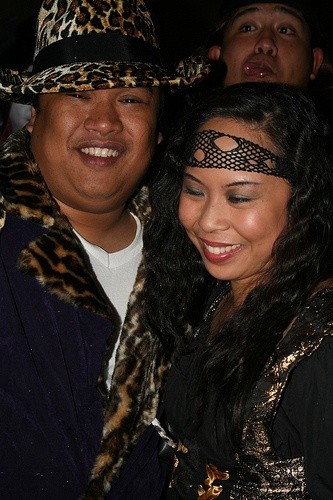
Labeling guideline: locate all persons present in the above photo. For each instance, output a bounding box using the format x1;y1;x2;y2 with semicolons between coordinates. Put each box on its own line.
0;0;220;500
85;78;333;500
207;0;324;90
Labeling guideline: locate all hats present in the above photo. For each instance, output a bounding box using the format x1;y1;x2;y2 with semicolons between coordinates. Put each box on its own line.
2;1;210;100
